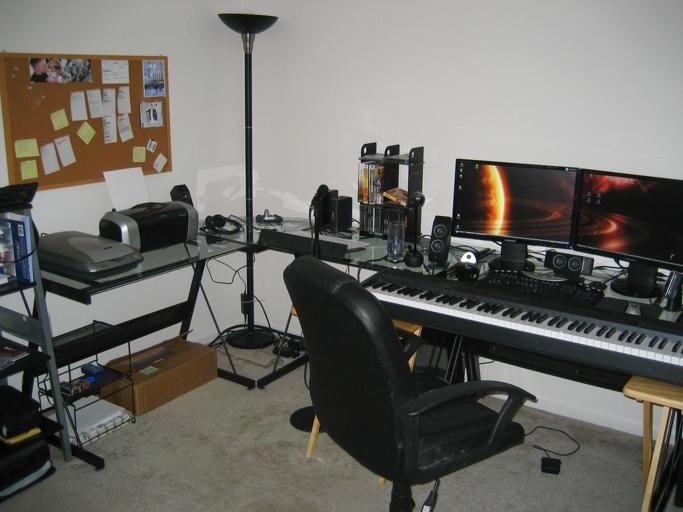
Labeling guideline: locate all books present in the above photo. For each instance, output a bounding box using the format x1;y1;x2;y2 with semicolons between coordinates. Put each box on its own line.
47;394;131;449
357;159;409;238
0;211;55;504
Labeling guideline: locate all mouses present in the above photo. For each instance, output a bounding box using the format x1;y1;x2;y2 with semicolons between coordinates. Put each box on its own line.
624;301;642;320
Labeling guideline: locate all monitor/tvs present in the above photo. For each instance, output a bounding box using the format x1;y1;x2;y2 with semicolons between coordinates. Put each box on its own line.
572;166;683;298
450;158;580;269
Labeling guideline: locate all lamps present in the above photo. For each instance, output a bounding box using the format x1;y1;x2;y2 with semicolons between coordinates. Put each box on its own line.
211;9;280;350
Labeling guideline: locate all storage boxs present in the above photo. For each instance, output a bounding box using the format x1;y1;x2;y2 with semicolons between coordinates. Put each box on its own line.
93;329;220;415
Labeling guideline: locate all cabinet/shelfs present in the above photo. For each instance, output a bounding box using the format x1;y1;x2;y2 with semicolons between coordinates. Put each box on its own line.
32;318;137;452
0;204;74;464
355;140;425;245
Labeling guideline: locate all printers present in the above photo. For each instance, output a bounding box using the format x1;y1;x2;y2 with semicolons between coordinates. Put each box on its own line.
99;200;199;253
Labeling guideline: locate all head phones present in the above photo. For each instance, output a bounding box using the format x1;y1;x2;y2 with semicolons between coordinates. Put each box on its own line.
206;214;242;235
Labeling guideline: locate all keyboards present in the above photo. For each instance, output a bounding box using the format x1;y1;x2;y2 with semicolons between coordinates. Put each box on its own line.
488;266;603;305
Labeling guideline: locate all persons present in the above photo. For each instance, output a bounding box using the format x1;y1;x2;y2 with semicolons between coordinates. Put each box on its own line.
145;105;158;124
28;56;93;84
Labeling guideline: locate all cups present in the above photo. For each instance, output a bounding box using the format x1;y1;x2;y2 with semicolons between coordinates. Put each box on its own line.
386;219;403;260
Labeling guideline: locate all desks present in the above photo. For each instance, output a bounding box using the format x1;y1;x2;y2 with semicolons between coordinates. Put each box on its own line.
22;213;683;409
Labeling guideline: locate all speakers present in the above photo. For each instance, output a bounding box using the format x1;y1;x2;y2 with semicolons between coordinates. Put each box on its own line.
170;184;195;208
543;250;594;276
428;216;451;263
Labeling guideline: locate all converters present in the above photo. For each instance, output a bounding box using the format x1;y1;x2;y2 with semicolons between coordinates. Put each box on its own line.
541;456;561;475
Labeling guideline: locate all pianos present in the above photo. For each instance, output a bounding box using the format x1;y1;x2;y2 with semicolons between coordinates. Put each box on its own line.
359;269;683;386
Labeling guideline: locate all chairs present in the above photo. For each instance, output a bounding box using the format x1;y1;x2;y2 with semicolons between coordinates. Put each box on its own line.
278;252;538;510
